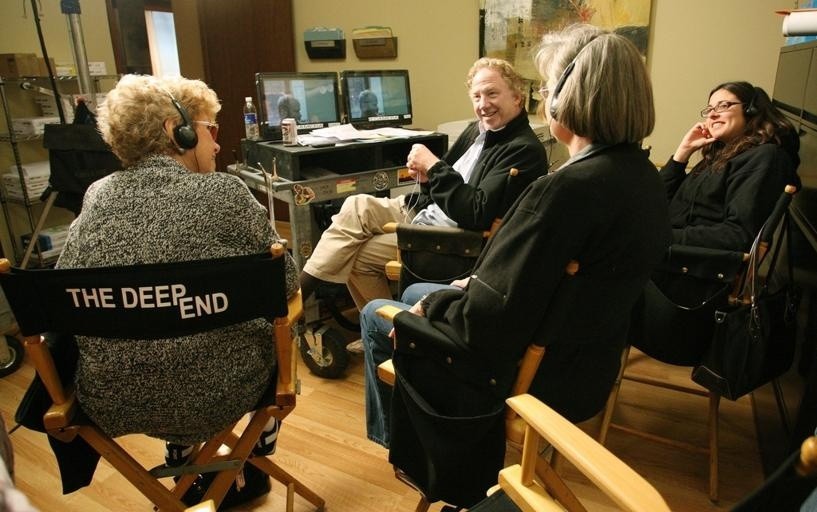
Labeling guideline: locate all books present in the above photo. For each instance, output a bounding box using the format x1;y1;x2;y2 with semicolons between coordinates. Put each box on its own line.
4;88;112;202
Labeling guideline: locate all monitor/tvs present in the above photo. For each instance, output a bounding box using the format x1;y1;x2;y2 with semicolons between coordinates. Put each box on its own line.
255;72;341;143
341;70;412;130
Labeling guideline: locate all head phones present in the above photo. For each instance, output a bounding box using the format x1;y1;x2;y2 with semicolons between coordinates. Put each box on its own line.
550;33;609;121
745;87;760;117
155;81;199;148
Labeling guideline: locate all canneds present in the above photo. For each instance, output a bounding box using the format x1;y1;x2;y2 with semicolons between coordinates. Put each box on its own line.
281;118;297;146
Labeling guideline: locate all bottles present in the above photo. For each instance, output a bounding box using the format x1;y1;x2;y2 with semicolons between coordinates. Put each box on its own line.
242;95;261;142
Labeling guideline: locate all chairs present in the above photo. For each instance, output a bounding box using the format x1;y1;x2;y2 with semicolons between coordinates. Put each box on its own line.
1;240;326;512
376;167;817;512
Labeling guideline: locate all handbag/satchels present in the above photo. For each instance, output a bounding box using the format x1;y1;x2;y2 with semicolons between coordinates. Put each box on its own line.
38;99;126;214
172;461;272;512
687;209;797;402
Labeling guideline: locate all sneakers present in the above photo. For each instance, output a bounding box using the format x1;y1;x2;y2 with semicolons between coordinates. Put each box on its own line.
345;337;366;355
251;417;281;457
163;439;194;468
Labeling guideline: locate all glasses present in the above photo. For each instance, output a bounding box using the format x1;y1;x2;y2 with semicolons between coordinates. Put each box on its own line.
700;99;744;119
187;117;220;141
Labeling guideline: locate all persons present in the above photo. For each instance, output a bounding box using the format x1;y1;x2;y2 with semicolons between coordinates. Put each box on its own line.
299;56;547;352
277;96;302;125
359;90;378;118
50;75;299;506
360;22;665;503
630;81;802;365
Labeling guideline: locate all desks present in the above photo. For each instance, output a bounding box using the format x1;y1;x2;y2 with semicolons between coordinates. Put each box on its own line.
227;163;421;380
